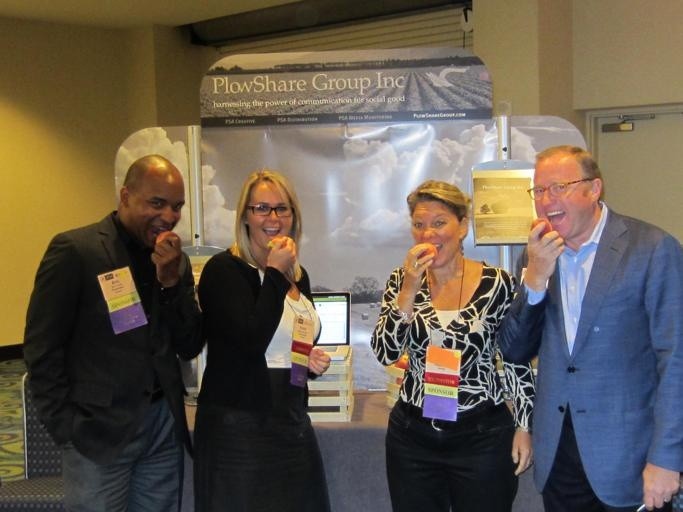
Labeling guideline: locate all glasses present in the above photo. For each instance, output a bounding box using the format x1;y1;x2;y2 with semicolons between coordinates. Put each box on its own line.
246;204;294;217
527;179;588;199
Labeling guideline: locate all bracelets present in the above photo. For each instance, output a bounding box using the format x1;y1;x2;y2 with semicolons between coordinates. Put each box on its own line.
395;306;412;321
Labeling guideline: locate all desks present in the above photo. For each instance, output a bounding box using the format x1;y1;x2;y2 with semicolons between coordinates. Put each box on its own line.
179;388;544;512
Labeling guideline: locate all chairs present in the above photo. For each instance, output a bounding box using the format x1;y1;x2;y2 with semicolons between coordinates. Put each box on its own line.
1;372;66;512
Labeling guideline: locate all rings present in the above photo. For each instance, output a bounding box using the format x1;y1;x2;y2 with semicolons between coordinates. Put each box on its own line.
413;261;420;268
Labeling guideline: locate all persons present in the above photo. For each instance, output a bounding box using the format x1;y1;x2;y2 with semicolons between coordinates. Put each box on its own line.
498;146;683;511
370;179;537;512
21;154;214;512
194;166;332;512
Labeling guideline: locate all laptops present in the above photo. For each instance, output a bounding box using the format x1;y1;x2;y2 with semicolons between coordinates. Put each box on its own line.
310;290;351;361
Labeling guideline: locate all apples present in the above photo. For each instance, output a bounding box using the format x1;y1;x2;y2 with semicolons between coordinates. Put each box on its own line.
531;218;554;239
417;243;437;264
157;231;181;246
267;238;287;253
394;355;410;370
